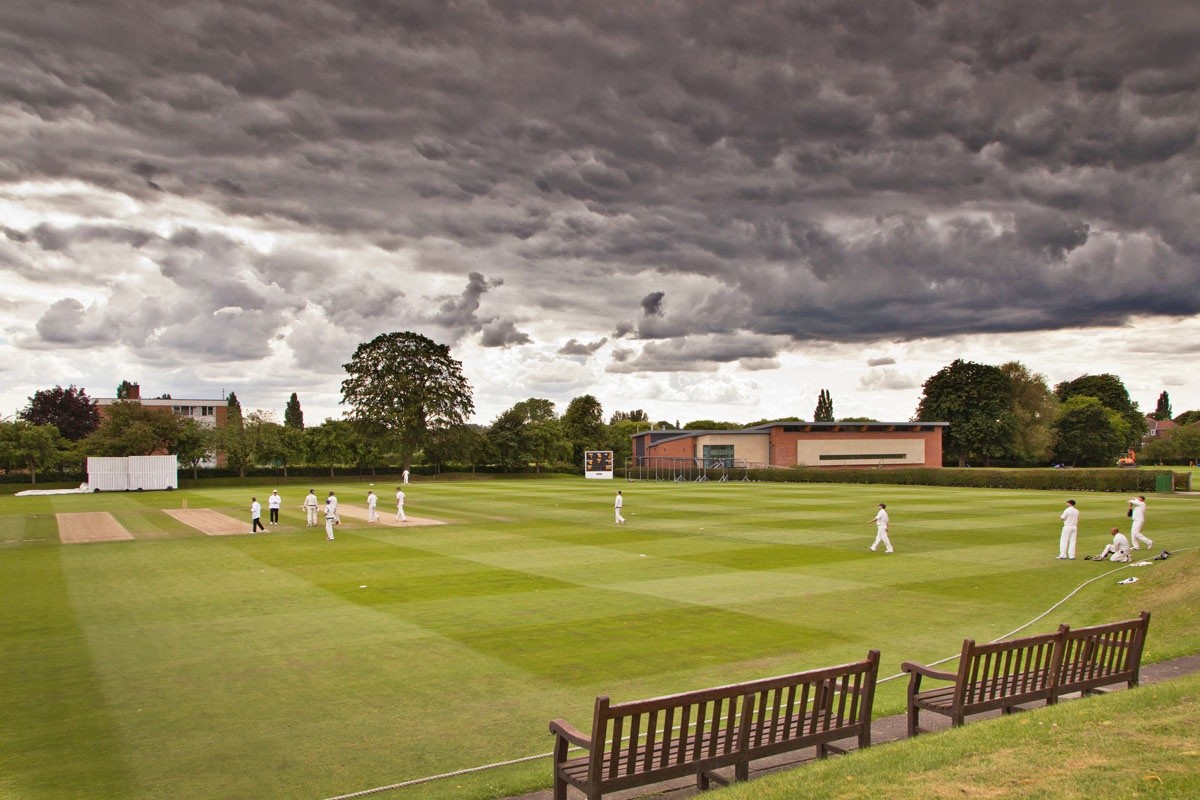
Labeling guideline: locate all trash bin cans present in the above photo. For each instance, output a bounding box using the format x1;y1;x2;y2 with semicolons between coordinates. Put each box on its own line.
1155;473;1172;493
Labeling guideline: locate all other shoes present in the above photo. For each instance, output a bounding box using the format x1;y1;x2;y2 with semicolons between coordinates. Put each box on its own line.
869;547;876;552
1148;541;1153;549
1069;557;1076;560
885;549;894;553
1056;556;1067;560
1130;547;1140;550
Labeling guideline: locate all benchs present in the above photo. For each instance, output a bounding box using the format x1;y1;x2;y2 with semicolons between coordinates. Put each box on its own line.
548;649;882;800
901;610;1150;738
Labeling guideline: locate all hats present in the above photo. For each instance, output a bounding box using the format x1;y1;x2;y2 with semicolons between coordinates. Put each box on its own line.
1066;500;1076;505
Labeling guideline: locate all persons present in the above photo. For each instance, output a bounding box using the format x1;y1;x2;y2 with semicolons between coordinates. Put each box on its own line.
1127;496;1152;550
269;490;281;526
868;504;894;553
367;491;380;523
396;487;407;522
1056;500;1079;560
615;490;625;526
303;489;318;527
251;497;266;535
323;491;340;540
402;467;410;485
1091;527;1129;561
